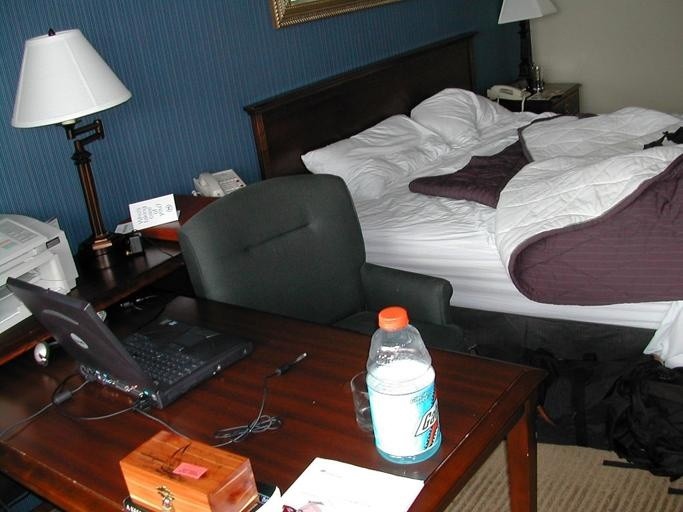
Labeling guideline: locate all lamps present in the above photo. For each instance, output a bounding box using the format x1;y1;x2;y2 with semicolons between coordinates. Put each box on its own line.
11;27;133;270
498;1;557;90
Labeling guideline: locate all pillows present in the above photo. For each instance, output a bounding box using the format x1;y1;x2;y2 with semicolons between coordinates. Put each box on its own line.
299;113;463;201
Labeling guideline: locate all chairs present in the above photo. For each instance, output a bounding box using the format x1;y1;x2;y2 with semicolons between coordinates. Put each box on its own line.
178;172;465;353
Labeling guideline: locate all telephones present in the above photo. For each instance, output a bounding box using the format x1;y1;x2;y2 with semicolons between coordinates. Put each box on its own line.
487;85;532;101
195;169;246;197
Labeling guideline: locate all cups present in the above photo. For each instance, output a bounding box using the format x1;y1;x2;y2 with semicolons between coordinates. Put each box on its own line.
349;369;373;434
531;66;544;87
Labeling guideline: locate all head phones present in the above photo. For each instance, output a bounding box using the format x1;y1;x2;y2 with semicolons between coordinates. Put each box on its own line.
34;340;60;367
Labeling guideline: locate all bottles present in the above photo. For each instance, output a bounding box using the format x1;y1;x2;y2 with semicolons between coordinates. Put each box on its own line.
366;305;445;466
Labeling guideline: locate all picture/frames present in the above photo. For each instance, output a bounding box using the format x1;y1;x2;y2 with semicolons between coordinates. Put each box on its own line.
268;0;396;30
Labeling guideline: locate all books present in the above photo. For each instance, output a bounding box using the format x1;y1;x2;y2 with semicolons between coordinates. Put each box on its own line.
123;481;281;512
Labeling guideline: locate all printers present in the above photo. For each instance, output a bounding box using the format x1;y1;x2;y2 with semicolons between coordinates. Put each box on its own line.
0;212;77;338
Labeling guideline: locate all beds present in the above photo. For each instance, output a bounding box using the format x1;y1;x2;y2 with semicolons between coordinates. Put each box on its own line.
242;21;683;448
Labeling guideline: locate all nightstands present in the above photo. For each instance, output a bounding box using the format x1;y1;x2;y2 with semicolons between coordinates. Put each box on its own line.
493;82;583;116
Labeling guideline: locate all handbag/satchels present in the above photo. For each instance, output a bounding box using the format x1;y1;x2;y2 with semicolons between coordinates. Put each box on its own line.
605;355;683;484
478;337;616;441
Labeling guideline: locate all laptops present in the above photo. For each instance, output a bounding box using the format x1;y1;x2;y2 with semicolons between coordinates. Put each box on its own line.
6;277;256;409
